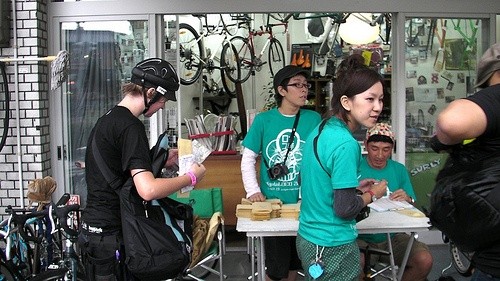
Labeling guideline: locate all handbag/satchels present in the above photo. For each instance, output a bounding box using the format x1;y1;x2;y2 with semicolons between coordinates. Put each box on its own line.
122;133;193;279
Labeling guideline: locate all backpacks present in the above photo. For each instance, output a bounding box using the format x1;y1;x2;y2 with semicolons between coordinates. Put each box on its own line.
429;88;500;251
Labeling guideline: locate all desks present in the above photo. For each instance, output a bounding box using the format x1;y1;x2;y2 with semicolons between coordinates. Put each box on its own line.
236;196;433;281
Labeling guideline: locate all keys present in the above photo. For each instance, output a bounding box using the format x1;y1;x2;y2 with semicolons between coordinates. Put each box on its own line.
306;262;325;281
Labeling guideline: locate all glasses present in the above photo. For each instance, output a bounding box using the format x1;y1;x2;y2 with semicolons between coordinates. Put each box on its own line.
284;83;312;89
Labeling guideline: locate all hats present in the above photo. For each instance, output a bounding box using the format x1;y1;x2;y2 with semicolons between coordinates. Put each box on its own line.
365;122;396;146
473;42;500;88
273;66;311;90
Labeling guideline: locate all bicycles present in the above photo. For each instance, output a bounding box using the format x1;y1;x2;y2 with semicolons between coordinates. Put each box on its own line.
165;12;242;98
1;192;82;281
222;19;289;82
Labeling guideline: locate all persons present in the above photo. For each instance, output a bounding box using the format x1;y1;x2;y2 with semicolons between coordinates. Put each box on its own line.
356;122;433;281
436;43;500;281
77;58;208;281
295;53;386;281
240;65;322;281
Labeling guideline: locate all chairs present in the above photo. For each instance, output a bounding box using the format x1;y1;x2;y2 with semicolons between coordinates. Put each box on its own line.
168;187;228;281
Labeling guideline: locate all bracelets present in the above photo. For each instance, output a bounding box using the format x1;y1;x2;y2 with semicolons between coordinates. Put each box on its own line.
187;172;196;185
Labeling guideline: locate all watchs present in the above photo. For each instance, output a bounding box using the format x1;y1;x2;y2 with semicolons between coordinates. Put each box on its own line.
410;196;415;204
365;190;376;203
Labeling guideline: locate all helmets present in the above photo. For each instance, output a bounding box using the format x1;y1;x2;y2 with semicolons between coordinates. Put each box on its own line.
131;58;179;100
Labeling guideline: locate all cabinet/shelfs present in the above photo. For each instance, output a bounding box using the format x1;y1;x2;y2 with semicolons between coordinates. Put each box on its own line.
301;76;391;118
